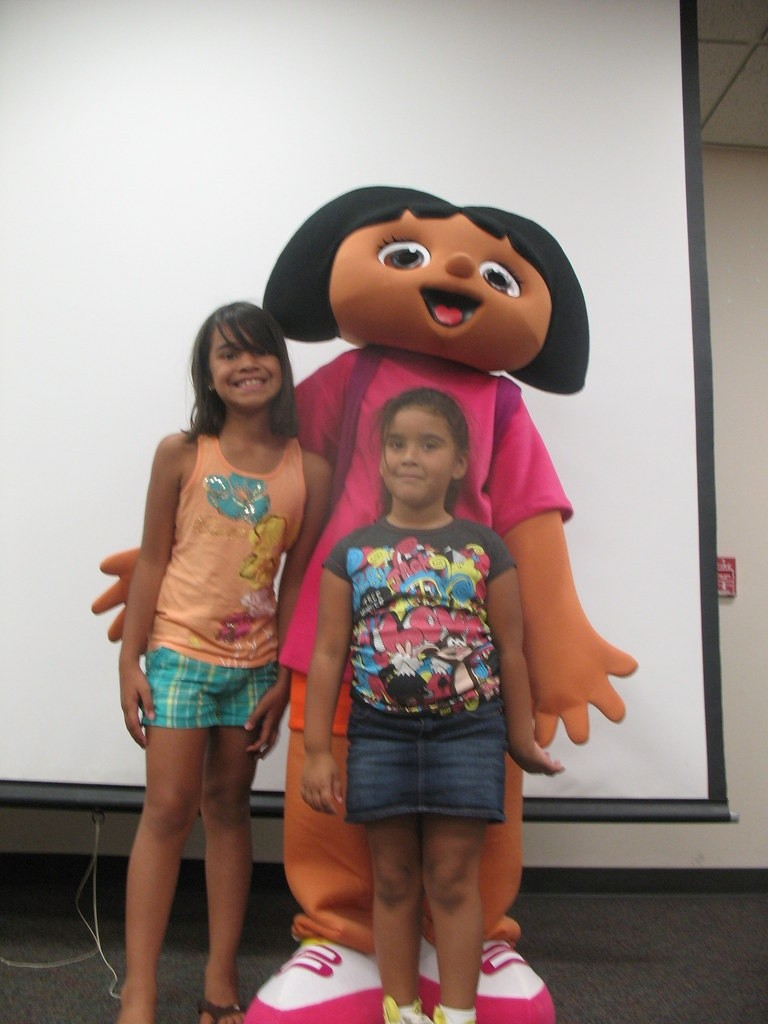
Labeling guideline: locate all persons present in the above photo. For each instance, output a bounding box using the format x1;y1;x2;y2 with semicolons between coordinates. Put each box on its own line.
92;185;638;1024
300;387;568;1024
118;300;328;1024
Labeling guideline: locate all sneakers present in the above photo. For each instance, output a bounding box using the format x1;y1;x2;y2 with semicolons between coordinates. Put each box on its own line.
383;993;434;1024
434;1005;476;1024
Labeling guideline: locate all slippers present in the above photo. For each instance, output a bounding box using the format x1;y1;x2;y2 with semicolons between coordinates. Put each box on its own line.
198;999;243;1024
